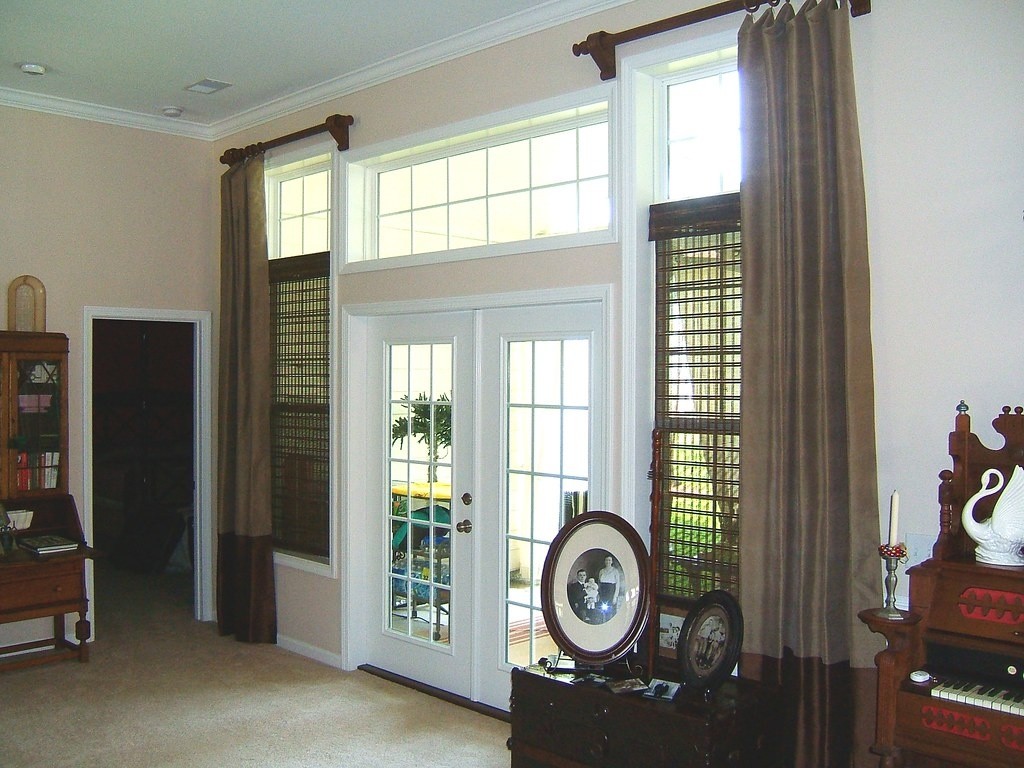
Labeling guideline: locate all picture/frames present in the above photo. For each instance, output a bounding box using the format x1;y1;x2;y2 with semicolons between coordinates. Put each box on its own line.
540;510;652;666
650;592;696;669
677;588;744;696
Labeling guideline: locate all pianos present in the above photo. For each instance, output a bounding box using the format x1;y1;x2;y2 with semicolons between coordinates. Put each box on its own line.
860;402;1024;767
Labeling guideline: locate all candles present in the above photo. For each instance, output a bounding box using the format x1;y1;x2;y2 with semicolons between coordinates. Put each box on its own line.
888;490;899;547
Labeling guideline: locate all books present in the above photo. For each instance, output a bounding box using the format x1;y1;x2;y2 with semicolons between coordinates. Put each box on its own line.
18;451;59;490
20;535;79;556
19;393;52;413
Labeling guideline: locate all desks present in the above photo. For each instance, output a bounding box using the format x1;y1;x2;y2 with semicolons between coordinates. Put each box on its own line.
389;482;451;502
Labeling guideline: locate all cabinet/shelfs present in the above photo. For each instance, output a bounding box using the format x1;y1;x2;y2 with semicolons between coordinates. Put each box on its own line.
390;540;451;640
506;654;784;768
0;329;106;675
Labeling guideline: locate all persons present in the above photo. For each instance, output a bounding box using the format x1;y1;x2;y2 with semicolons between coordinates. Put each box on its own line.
655;683;668;696
667;623;680;646
696;619;724;662
570;555;621;621
